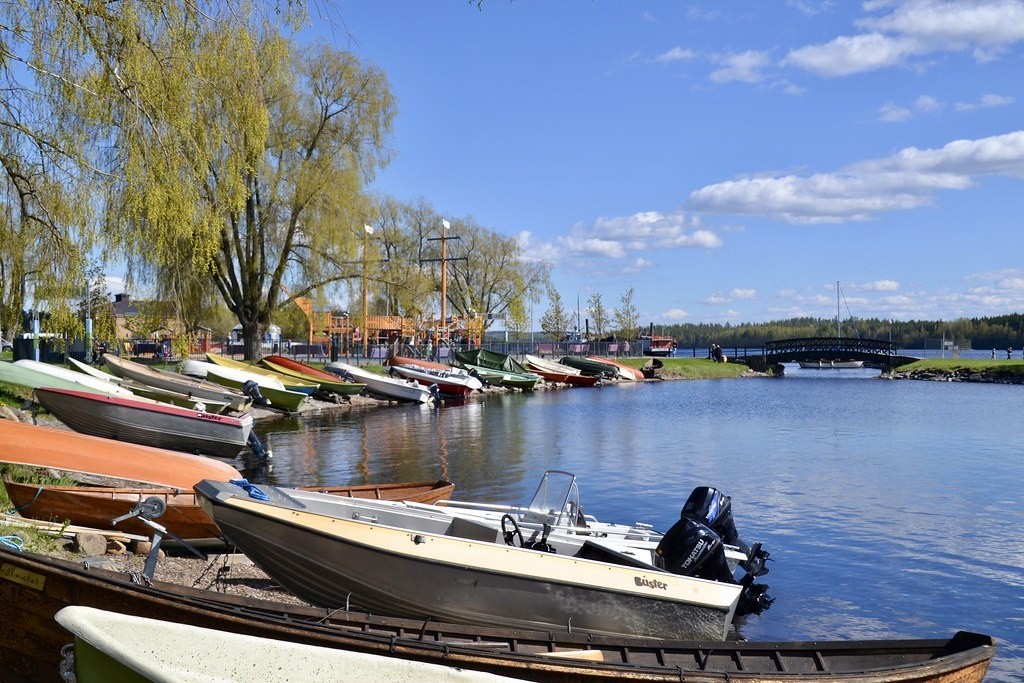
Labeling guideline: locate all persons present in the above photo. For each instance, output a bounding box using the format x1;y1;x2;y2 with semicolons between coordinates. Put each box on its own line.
622;341;630;357
672;339;678;357
991;347;996;360
1007;347;1012;360
710;344;722;363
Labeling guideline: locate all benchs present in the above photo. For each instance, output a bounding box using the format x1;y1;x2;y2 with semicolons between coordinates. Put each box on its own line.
428;348;455;364
134;344;161;357
536;344;559;359
569;344;590;355
292;345;323;362
227;345;244;360
608;344;630;357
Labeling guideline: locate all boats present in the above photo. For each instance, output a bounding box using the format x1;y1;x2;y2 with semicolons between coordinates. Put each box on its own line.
195;468;778;642
35;387;271;462
0;419;243;490
55;605;535;682
0;353;645;415
4;472;456;540
0;541;998;682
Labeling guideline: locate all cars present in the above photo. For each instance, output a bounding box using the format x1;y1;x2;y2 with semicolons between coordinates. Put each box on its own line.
1;338;13;352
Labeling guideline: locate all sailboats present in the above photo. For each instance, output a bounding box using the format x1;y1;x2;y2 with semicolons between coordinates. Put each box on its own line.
797;281;865;369
